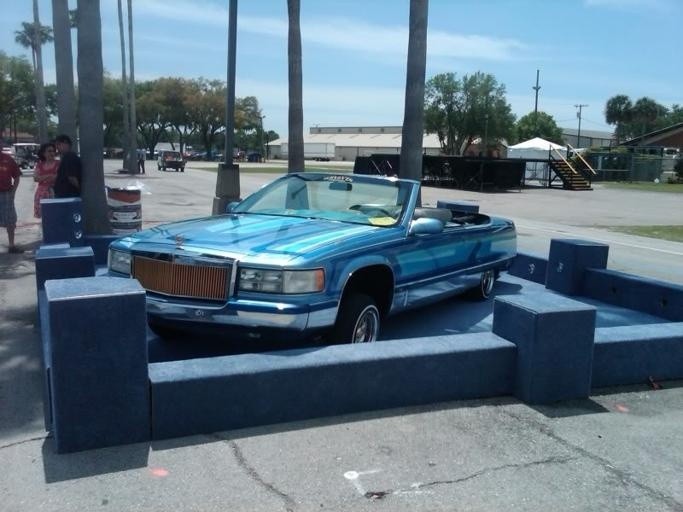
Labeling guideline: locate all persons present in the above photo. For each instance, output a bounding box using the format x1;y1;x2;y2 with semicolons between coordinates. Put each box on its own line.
32;142;60;218
0;137;26;254
138;144;146;174
48;133;83;198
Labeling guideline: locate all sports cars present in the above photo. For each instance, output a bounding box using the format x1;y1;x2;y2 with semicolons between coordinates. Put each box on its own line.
106;171;518;345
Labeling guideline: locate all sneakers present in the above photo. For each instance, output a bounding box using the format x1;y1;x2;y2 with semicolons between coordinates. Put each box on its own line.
8;246;26;254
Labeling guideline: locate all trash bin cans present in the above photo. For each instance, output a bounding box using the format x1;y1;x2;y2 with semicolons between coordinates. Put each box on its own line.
107;186;142;236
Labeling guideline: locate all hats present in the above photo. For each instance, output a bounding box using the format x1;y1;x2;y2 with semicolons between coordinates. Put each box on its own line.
49;135;73;144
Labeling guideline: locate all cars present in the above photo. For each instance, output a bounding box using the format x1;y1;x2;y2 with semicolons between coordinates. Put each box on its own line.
185;150;246;163
157;150;185;171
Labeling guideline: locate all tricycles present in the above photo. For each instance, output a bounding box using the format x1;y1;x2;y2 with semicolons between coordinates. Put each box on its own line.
8;142;42;169
